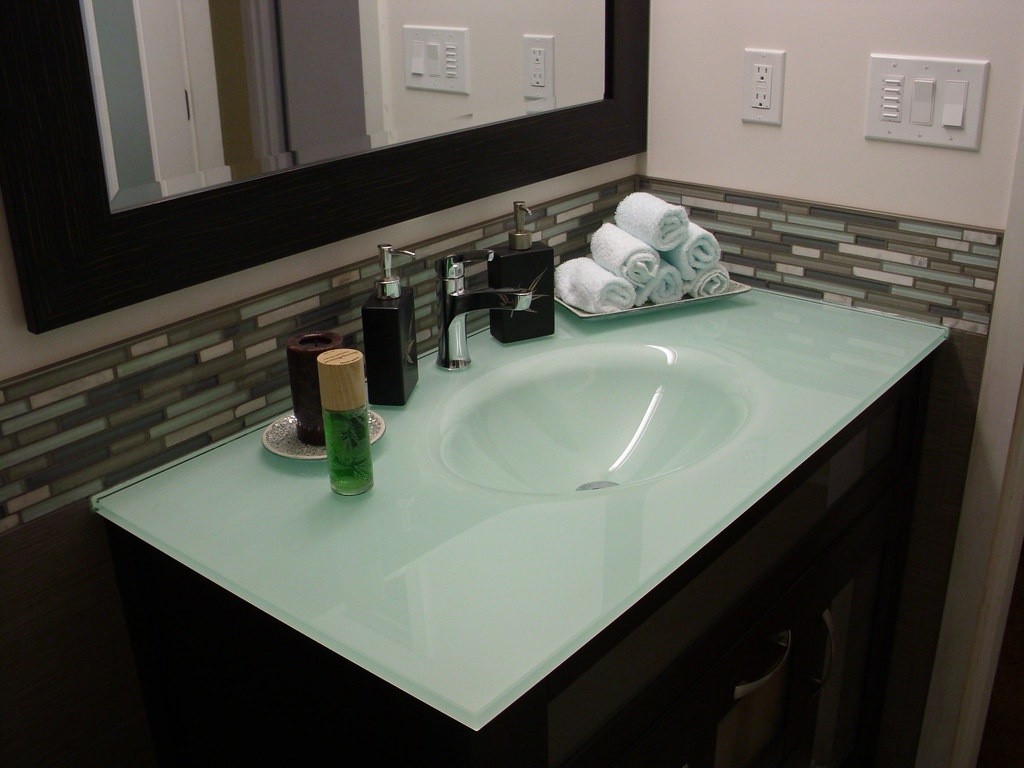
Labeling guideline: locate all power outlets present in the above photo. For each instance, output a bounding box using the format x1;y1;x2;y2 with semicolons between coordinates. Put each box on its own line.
740;47;787;126
521;33;556;99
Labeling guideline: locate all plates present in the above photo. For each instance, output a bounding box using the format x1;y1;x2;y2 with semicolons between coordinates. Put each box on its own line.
261;411;385;459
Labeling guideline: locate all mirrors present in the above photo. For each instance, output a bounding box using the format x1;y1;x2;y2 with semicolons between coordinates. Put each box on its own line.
0;0;650;336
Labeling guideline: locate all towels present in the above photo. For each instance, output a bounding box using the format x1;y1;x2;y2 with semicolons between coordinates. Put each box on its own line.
554;192;730;313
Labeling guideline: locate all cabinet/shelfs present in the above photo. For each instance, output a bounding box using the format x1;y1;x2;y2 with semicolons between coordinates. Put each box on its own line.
104;351;947;767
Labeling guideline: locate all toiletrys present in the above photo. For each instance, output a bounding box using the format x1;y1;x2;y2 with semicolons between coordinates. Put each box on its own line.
317;349;374;496
362;244;418;406
487;201;555;344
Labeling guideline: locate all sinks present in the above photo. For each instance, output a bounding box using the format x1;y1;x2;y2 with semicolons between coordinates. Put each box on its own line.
424;334;778;504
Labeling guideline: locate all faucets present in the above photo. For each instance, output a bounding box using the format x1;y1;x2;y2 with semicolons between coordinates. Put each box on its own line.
434;249;532;372
